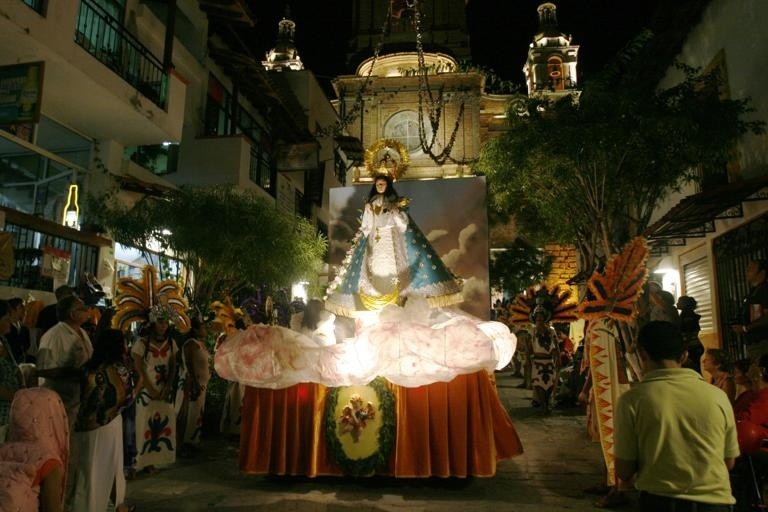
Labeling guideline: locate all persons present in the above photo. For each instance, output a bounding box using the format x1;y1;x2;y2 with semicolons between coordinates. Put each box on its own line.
359;171;411;307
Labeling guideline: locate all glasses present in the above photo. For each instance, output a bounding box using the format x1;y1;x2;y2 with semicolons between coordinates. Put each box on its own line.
72;304;90;313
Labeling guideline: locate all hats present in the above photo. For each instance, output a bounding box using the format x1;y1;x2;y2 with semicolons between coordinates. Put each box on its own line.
148;303;172;323
